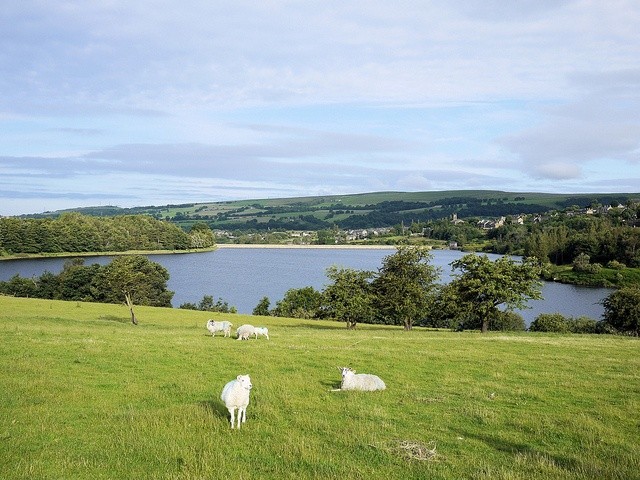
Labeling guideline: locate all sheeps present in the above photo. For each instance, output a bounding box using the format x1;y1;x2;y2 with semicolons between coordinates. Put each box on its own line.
328;366;386;392
220;374;253;430
234;324;255;340
207;319;233;337
254;327;269;339
